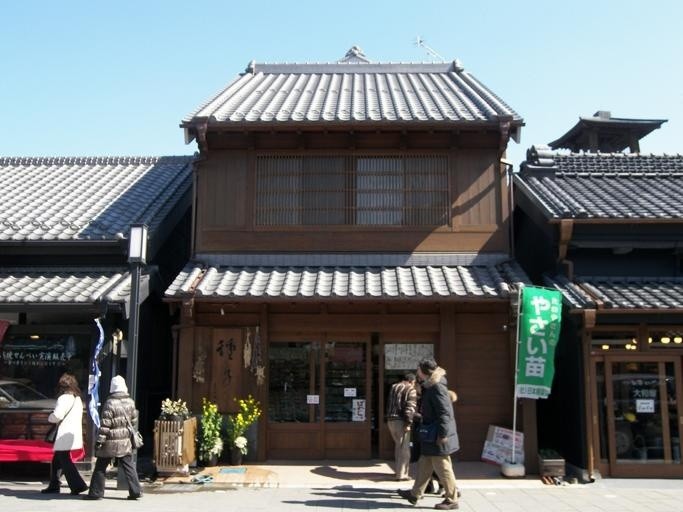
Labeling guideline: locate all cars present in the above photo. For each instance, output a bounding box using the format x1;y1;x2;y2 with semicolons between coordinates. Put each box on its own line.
0;381;87;412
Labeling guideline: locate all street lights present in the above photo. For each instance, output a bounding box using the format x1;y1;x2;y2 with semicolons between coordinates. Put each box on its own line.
117;223;149;490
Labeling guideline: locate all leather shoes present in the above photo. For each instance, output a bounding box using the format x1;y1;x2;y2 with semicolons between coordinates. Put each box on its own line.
41;485;60;493
395;476;414;481
70;485;88;495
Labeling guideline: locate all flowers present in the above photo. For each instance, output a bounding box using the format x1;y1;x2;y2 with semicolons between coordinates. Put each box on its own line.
194;393;262;462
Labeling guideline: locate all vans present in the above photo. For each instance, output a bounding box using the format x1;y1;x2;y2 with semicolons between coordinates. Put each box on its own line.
602;374;675;458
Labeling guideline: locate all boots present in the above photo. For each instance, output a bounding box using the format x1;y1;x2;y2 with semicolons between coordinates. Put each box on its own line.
435;499;459;510
395;486;420;506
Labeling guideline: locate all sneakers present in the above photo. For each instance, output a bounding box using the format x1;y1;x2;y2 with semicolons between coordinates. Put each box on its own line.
83;495;99;500
127;492;140;500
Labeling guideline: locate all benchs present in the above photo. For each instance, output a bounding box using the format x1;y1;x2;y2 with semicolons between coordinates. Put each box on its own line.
0;411;87;483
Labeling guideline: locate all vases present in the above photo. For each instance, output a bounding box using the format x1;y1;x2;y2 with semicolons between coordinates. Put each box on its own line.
202;452;219;467
229;447;243;465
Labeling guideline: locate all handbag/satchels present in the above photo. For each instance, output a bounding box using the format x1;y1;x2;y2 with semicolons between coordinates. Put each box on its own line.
415;424;436;443
45;423;59;443
132;429;144;448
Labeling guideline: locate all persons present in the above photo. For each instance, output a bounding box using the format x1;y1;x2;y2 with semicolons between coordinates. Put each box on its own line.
41;374;88;495
397;355;461;510
83;375;144;498
386;370;417;481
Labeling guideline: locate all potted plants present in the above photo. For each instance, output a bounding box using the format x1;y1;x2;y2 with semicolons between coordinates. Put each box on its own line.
536;448;566;477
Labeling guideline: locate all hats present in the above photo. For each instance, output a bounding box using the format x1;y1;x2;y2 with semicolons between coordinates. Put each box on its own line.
109;375;128;393
